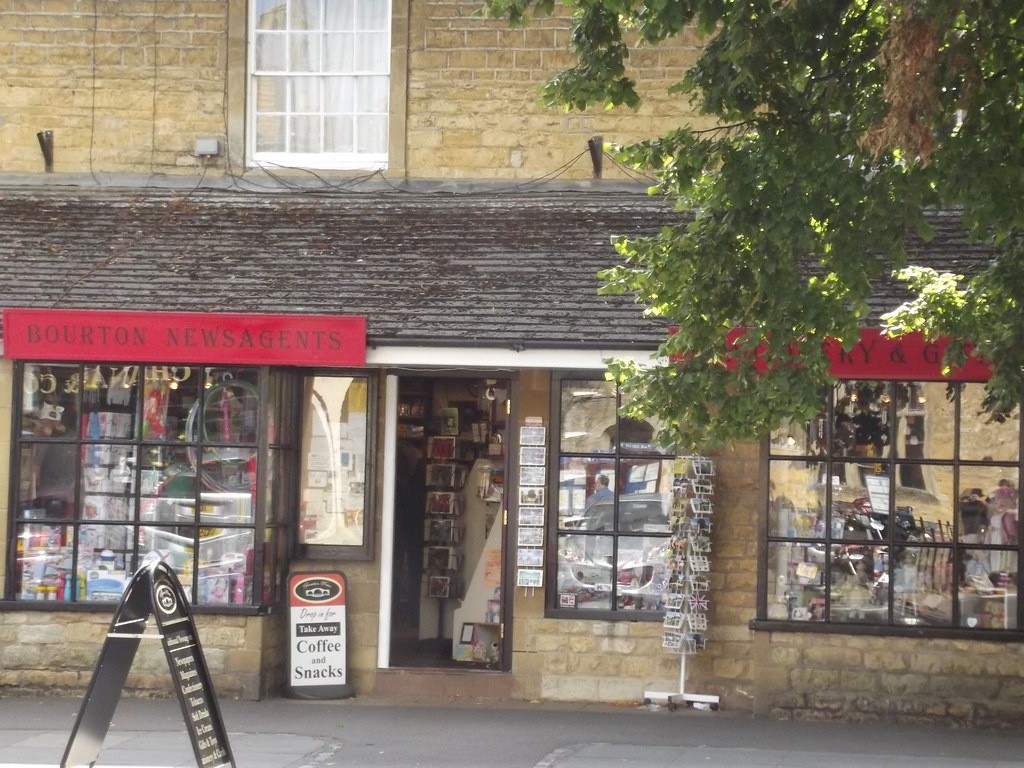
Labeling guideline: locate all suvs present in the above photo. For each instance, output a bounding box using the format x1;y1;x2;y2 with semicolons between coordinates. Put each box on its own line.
140;447;261;567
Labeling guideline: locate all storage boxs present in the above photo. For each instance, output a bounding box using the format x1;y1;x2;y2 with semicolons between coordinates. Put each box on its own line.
17;494;257;601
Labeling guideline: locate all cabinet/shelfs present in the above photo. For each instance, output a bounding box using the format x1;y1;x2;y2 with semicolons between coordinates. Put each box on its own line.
426;440;466;649
643;453;720;714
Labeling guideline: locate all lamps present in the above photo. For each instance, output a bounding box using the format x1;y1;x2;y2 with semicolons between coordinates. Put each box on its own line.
205;369;213;389
122;368;130;388
170;367;180;390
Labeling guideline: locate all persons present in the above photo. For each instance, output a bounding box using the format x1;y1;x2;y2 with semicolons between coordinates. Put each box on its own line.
943;545;996;595
30;420;72;500
834;556;879;619
585;475;615;561
898;550;921;616
985;479;1018;590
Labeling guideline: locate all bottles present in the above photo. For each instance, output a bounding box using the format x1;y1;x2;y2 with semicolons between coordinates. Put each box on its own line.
13;560;86;601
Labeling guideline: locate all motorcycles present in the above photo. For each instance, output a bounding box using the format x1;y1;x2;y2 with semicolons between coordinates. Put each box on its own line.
796;505;918;610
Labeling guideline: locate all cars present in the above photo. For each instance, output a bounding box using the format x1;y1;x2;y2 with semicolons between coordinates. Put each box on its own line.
555;492;675;609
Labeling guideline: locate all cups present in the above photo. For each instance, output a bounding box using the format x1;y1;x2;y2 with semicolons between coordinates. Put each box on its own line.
98;411;131;439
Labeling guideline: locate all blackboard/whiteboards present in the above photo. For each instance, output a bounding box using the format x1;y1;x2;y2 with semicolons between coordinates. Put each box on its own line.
60;561;237;768
